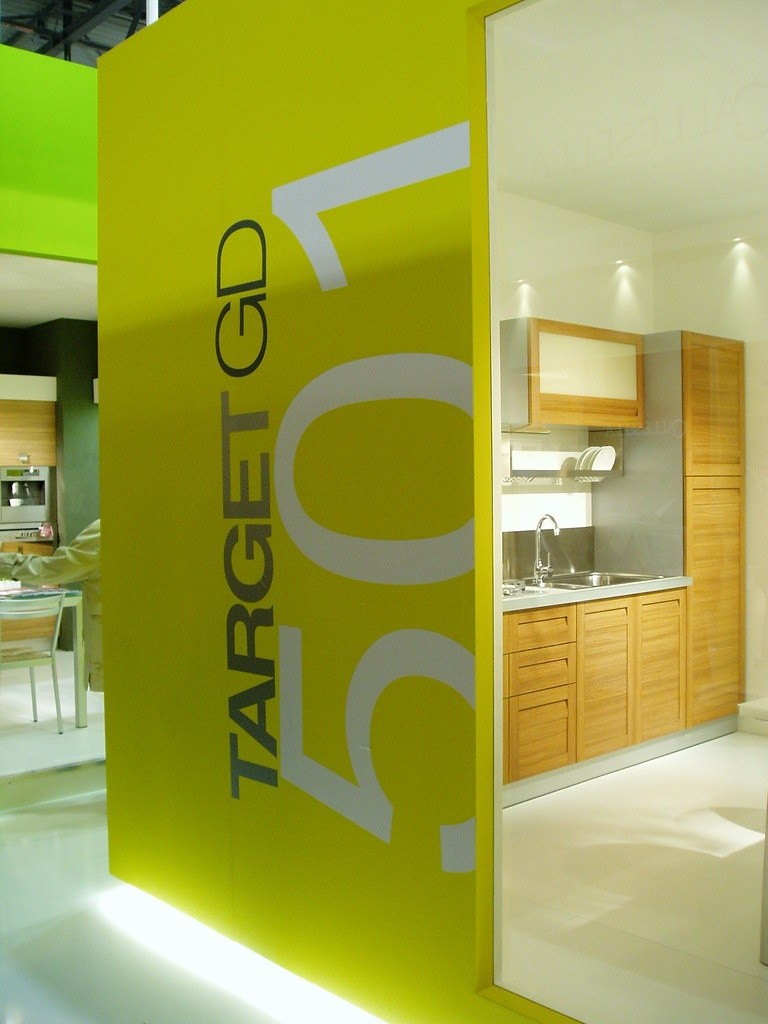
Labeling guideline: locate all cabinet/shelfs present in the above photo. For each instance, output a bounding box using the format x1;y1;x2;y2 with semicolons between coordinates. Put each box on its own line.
592;330;746;748
501;317;645;434
577;586;686;784
503;602;576;809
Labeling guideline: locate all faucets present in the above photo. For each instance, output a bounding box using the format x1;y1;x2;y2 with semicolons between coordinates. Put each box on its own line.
533;514;560;587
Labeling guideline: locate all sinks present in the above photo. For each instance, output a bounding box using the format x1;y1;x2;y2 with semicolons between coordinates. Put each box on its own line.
541;570;665;588
523;579;593;590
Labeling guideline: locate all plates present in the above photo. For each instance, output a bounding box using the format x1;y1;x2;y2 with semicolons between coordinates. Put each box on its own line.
575;446;615;482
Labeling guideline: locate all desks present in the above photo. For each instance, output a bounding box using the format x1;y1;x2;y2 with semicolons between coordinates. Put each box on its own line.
0;587;89;727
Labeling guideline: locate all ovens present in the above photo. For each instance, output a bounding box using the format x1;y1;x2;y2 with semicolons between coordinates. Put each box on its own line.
0;467;57;542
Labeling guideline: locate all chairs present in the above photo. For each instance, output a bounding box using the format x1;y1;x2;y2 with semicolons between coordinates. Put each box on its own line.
0;542;59;589
0;592;66;734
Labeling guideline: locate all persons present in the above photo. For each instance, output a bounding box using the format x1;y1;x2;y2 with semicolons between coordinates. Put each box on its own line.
0;517;104;693
